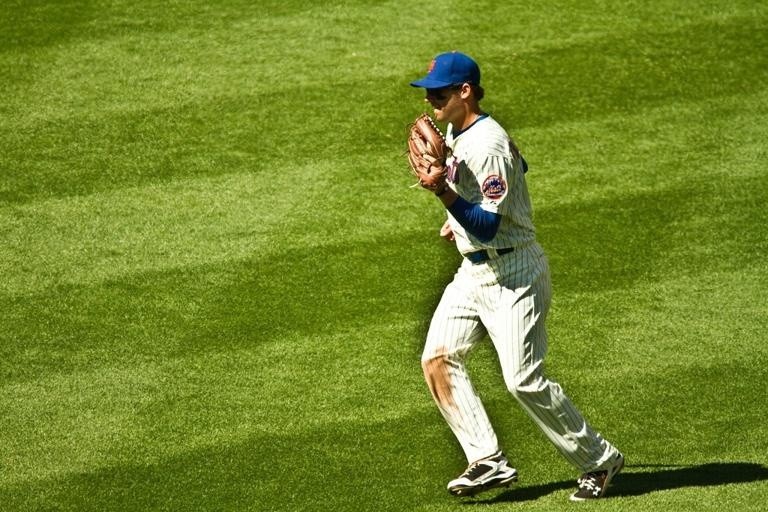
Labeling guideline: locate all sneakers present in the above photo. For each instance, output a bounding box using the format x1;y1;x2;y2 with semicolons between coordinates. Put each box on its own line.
447;449;519;498
570;452;624;500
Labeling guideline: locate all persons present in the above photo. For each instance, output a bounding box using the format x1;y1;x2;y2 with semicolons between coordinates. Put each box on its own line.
407;51;626;502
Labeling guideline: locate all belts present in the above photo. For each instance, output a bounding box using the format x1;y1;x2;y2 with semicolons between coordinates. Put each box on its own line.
464;248;513;263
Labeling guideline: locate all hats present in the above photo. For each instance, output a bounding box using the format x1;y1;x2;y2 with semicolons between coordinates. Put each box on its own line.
410;50;480;89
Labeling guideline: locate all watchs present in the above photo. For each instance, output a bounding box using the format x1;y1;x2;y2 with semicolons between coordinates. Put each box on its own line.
435;183;448;196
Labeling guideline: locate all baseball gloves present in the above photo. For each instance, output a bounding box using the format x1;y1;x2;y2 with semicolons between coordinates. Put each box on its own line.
406;113;449;190
